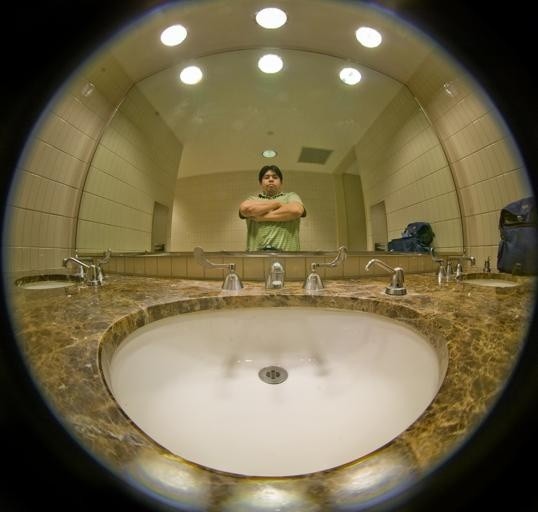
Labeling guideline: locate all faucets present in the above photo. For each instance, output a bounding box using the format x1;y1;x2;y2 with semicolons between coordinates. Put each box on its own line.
446;255;476;275
62;256;104;286
265;261;287;289
365;259;406;295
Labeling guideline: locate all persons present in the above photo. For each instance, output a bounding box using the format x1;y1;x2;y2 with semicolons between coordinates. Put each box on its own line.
238;165;307;251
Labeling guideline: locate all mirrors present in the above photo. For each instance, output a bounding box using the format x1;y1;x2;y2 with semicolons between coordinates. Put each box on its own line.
73;49;470;255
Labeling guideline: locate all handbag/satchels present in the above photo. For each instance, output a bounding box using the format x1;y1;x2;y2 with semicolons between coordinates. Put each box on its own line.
497;197;538;275
388;222;433;252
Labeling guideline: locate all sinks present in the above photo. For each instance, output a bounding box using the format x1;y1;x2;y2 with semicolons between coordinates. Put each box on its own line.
93;293;453;481
454;271;525;289
13;272;84;291
